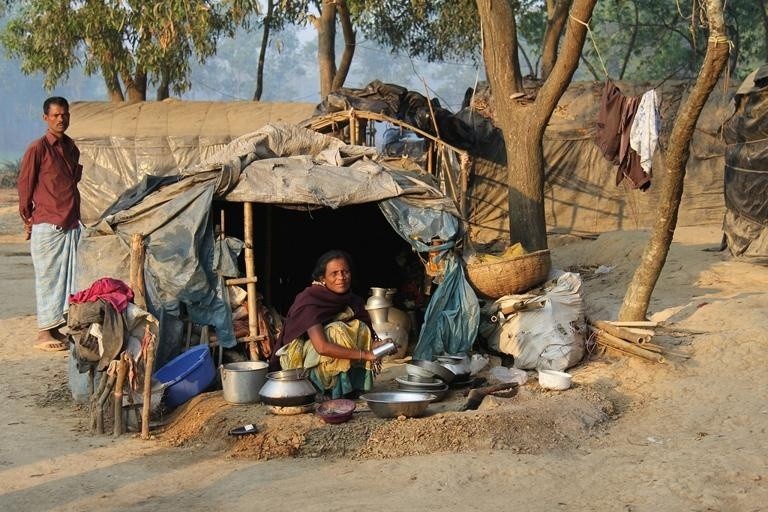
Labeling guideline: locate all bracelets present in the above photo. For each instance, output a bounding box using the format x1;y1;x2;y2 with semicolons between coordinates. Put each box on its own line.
359;348;363;362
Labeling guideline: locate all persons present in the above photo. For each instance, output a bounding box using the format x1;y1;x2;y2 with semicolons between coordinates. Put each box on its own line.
18;96;83;352
269;251;402;402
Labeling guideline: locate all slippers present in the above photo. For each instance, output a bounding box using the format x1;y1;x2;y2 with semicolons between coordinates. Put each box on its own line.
58;335;70;347
32;338;68;351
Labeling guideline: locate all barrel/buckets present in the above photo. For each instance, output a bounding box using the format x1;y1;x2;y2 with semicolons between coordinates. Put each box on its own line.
217;361;270;406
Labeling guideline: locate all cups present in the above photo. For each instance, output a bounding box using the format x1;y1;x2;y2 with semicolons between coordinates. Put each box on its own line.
372;341;396;357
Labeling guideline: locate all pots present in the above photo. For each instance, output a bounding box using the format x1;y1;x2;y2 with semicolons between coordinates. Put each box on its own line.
431;356;469;382
218;361;317;407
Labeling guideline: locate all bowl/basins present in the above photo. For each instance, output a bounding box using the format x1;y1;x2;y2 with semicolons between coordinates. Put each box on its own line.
537;369;572;391
316;360;456;424
150;343;217;409
